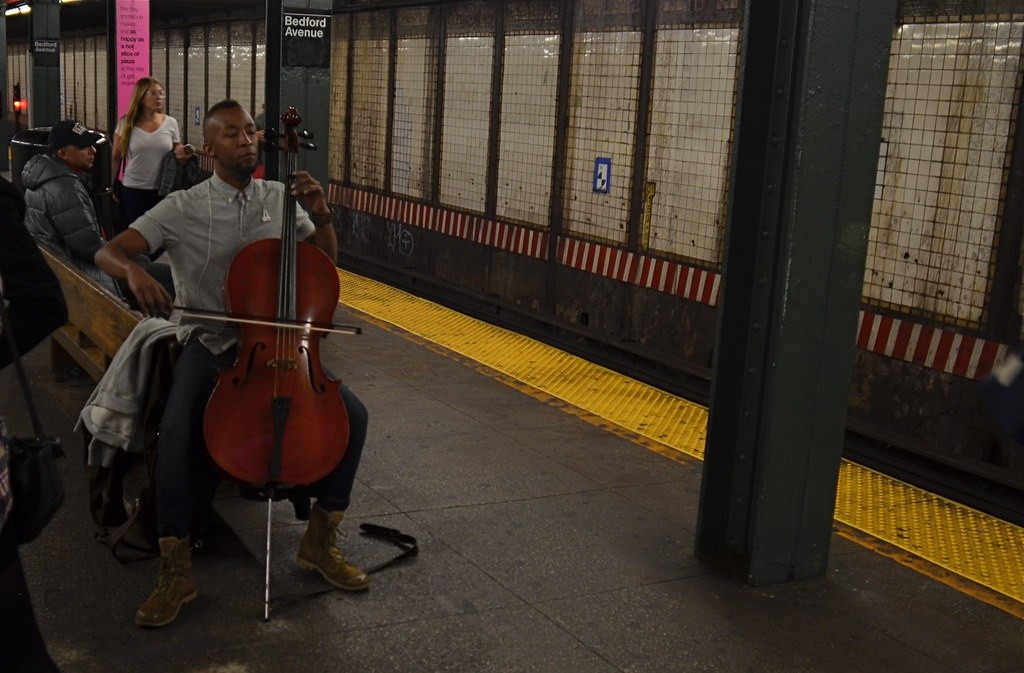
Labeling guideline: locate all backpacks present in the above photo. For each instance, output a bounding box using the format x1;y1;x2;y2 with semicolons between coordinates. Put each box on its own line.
90;426;158;564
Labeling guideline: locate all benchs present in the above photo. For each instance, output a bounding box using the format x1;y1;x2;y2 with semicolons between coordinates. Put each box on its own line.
37;239;142;383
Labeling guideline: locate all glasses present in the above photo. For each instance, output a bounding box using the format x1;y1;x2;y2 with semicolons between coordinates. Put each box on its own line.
144;89;166;99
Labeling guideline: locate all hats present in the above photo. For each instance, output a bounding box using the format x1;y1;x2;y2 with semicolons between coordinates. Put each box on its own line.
46;119;103;147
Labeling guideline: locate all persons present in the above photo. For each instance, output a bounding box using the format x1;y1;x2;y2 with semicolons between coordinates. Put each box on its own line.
111;77;193;259
22;120;176;310
95;100;371;626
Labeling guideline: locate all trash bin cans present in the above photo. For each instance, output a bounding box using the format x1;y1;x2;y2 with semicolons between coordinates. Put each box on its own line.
10;125;114;242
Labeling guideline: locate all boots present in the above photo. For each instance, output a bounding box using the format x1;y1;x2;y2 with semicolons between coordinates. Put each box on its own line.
134;534;199;626
293;501;370;591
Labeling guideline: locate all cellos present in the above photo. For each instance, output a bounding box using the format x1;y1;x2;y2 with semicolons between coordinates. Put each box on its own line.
200;100;356;625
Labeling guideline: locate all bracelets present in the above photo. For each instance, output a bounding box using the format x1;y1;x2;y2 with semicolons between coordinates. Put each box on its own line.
309;204;335;226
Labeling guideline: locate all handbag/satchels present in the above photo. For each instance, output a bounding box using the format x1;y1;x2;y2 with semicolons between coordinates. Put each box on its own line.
0;434;68;542
